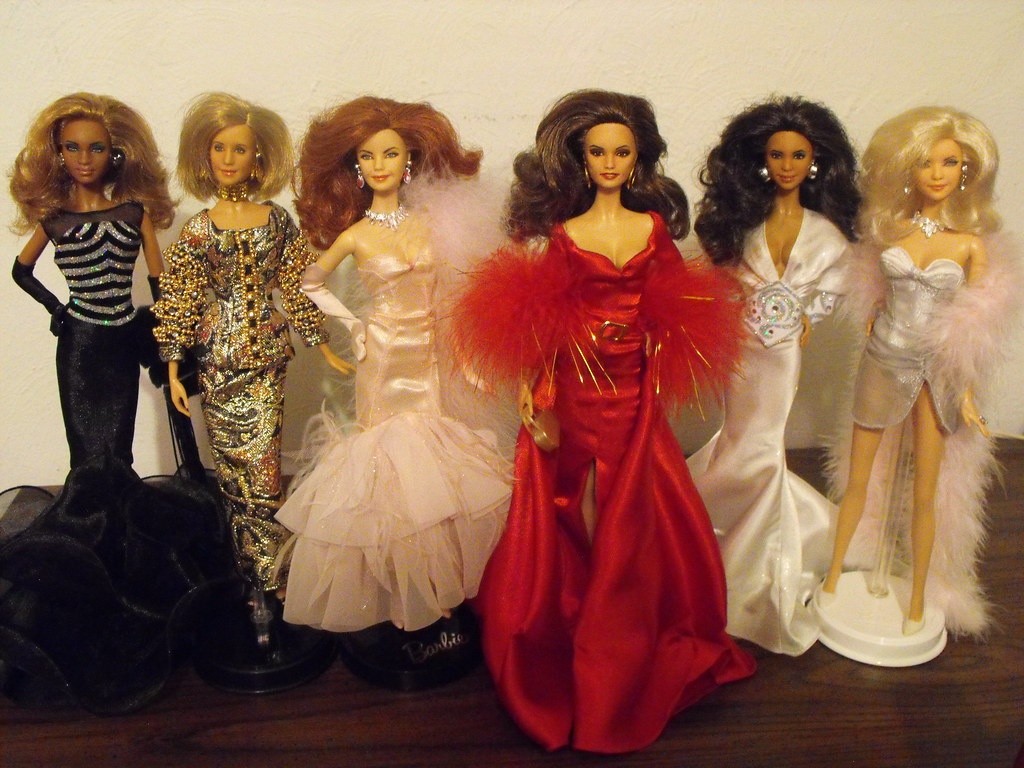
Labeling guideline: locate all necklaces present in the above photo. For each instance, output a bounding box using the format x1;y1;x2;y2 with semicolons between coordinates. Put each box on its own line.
361;203;410;231
911;206;948;237
216;181;251;202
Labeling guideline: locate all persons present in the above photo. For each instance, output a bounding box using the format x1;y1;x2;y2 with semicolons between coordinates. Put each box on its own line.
149;91;355;660
1;94;230;714
821;106;1011;636
275;95;512;658
680;96;879;658
455;90;757;755
